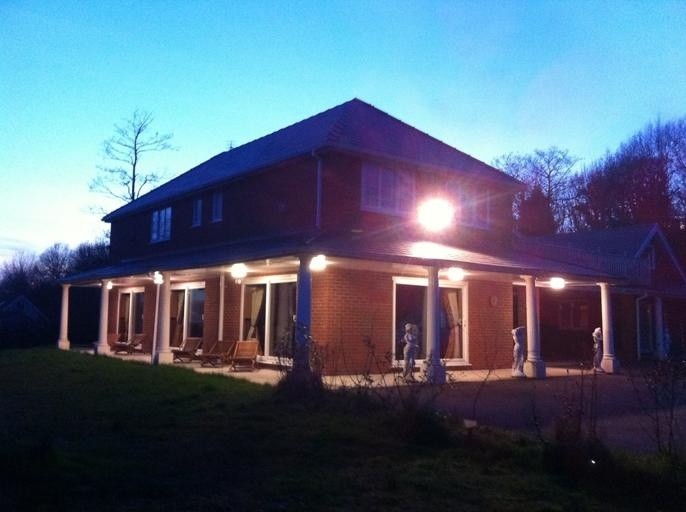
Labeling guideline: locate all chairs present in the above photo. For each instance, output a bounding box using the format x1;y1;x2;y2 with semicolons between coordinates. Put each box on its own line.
171;338;259;373
94;333;147;355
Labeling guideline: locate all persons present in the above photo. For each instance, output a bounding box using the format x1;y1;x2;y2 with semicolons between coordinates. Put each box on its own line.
591;328;604;372
402;324;419;381
509;326;523;375
